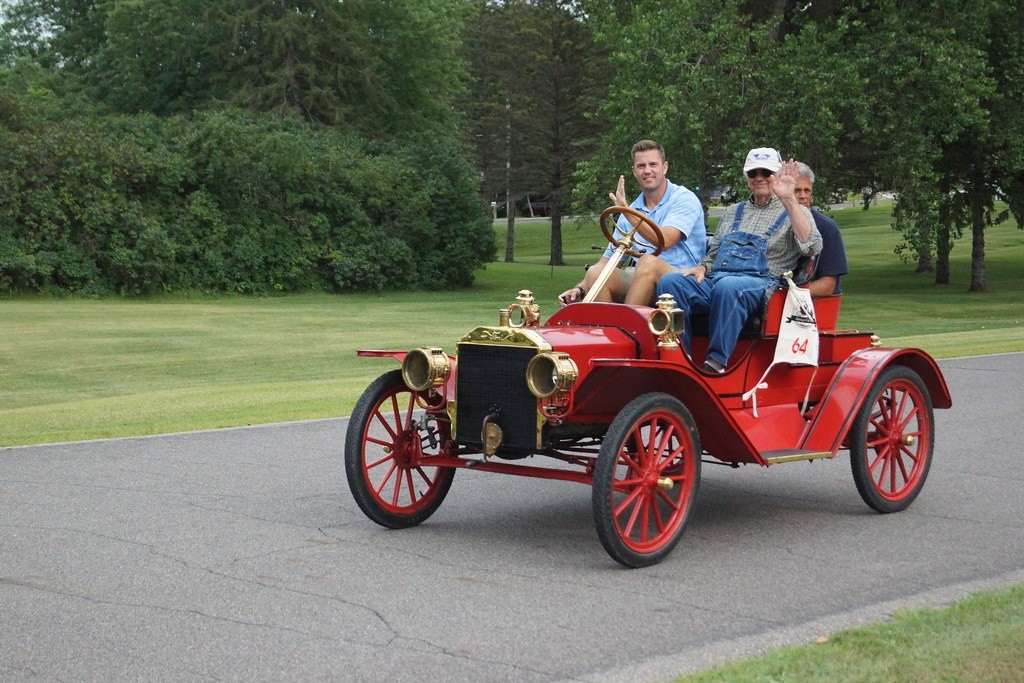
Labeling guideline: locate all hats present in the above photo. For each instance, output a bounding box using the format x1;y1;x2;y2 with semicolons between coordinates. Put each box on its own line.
743;148;782;177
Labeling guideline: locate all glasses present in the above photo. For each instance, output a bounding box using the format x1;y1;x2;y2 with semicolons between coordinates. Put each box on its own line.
747;168;773;178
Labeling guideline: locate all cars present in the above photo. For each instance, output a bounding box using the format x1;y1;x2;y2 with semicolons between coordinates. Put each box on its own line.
343;206;951;569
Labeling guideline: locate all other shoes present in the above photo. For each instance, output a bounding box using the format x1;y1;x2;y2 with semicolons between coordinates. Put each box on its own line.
703;359;725;373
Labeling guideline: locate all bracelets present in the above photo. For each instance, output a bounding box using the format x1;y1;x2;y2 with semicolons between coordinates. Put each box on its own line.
575;285;586;300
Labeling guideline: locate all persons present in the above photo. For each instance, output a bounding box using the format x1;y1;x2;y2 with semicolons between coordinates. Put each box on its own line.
775;159;850;300
557;139;707;307
656;147;825;373
